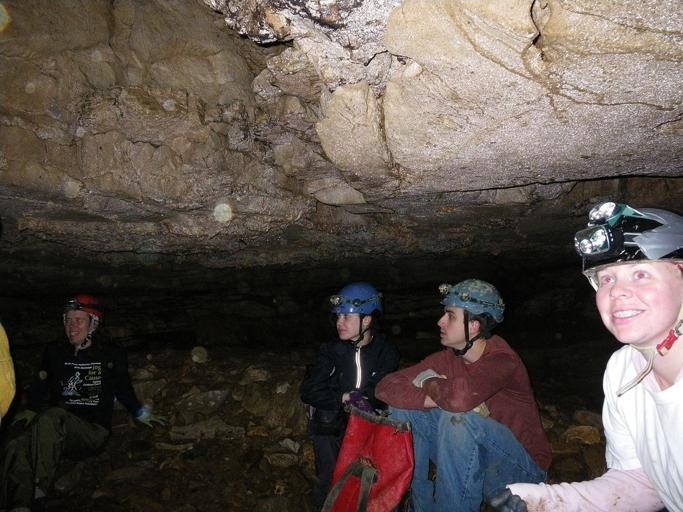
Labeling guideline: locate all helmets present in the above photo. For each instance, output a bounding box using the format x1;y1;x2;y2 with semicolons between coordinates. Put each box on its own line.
63;293;105;318
439;278;504;323
573;206;683;292
331;278;382;324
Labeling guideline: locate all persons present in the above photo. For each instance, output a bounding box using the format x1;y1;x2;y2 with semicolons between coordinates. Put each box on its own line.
298;284;402;511
484;202;682;512
2;296;169;511
375;276;552;512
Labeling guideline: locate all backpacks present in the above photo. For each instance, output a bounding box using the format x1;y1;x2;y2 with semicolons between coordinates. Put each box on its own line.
320;403;415;512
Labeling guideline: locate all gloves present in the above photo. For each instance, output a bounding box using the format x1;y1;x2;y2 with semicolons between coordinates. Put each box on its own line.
473;400;491;417
136;408;169;429
412;368;443;390
491;483;531;511
346;390;375;416
